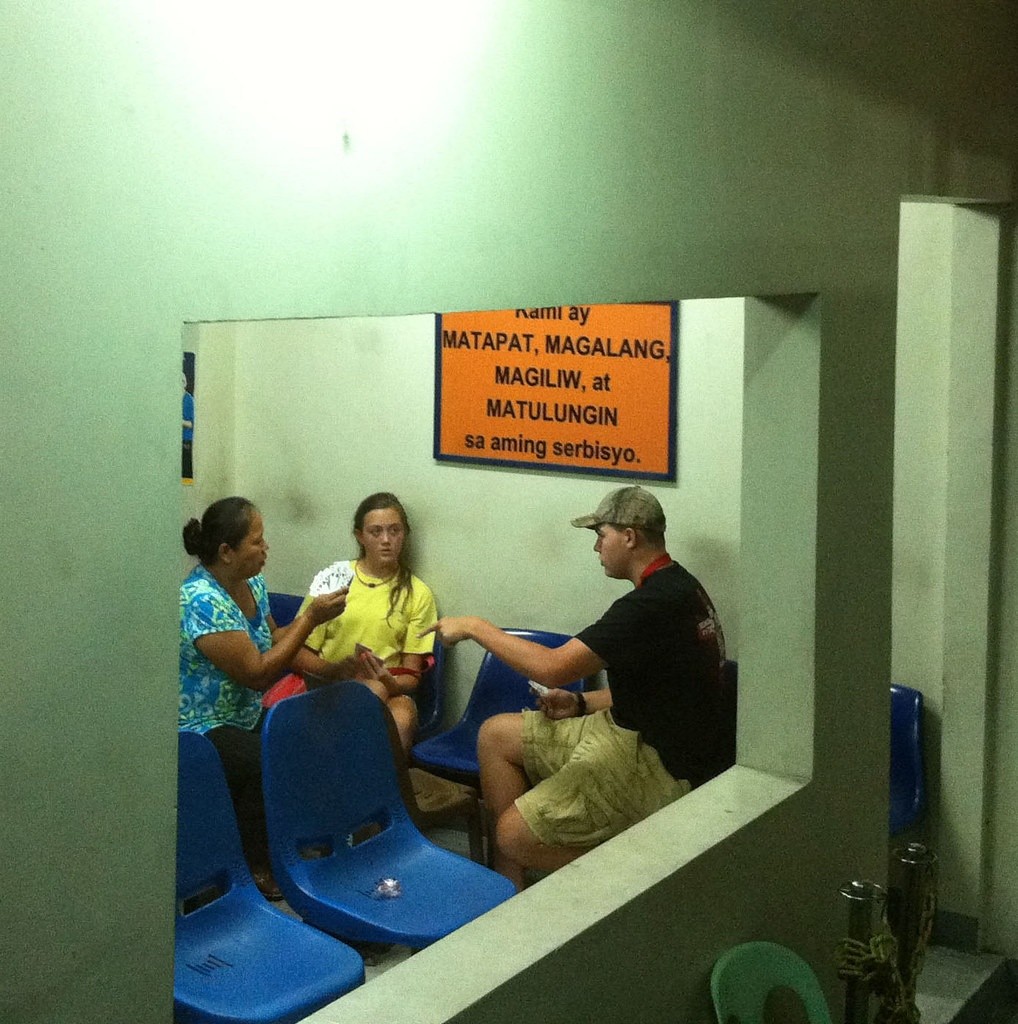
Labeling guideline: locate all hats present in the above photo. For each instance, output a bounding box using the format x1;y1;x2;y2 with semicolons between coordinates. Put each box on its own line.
571;485;666;530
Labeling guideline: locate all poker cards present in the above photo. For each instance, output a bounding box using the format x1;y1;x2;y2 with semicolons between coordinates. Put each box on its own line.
305;558;357;599
527;679;552;707
355;644;384;669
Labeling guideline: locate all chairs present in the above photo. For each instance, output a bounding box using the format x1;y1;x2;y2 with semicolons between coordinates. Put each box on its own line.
172;590;925;1024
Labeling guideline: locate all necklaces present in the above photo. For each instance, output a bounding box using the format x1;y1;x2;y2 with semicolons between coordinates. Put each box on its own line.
354;561;400;588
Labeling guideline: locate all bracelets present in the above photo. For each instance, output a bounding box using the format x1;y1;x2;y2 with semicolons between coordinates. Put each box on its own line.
575;690;587;716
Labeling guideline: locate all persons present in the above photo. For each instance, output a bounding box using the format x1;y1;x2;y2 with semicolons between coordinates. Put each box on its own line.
257;490;438;763
177;495;364;899
416;481;738;892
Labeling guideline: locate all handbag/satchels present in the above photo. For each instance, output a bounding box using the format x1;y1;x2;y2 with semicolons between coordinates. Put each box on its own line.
262;672;315;708
